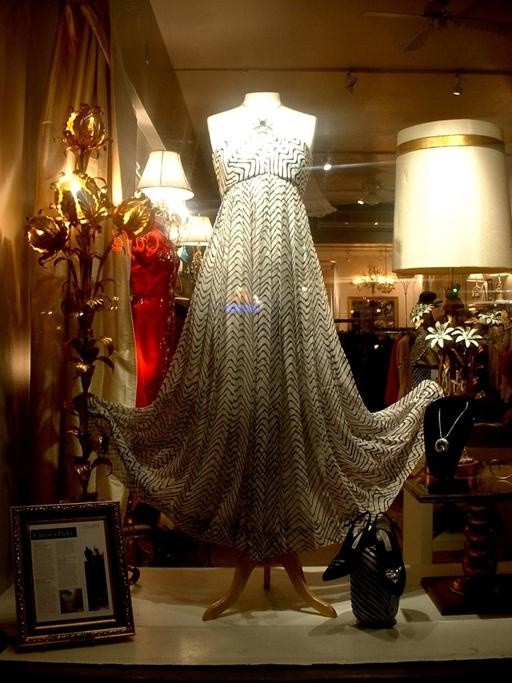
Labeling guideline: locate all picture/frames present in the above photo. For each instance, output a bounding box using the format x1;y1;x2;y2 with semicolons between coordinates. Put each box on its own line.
7;500;136;654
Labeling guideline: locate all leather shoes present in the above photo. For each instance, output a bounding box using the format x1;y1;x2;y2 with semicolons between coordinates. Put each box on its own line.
371;512;405;596
323;511;372;581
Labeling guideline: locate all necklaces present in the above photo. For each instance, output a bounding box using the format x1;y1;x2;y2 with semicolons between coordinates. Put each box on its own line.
432;397;469;452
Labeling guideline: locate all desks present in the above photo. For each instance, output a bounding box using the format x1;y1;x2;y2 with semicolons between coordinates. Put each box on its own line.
395;468;511;586
1;560;507;682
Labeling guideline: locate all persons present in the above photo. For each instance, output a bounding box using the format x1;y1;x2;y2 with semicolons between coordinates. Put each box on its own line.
128;211;182;408
201;87;322;563
413;290;440;327
435;297;467;327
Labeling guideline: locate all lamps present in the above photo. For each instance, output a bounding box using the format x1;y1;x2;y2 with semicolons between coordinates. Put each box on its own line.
393;117;511;306
140;149;215;291
